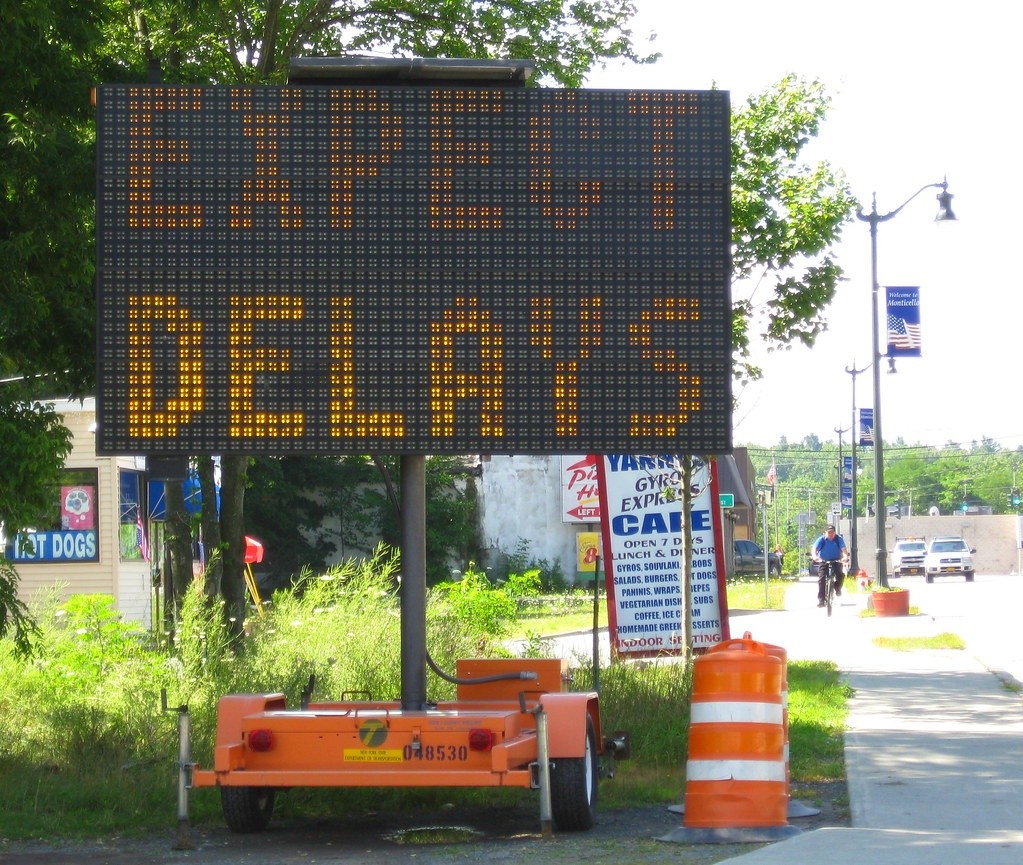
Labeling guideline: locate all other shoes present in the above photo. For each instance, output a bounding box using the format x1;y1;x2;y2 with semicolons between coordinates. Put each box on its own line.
835;587;841;596
817;599;825;606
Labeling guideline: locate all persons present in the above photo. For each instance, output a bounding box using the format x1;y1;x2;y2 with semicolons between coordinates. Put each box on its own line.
812;526;849;608
774;544;785;573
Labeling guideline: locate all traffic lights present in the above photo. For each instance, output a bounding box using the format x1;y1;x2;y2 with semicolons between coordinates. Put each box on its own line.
1010;487;1022;511
787;521;793;534
961;494;969;511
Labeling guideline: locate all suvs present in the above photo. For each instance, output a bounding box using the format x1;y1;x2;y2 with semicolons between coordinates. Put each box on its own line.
889;537;930;578
923;536;976;583
735;540;781;579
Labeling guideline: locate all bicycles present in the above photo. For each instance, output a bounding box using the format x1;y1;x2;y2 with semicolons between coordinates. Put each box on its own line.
813;558;849;617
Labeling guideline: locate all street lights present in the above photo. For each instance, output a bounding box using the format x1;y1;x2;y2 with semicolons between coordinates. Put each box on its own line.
833;457;863;502
846;349;897;577
855;176;959;589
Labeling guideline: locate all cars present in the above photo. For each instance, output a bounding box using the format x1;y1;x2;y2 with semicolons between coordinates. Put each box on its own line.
805;548;833;577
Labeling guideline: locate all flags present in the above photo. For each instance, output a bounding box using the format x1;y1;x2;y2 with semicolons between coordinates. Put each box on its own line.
136;516;148;560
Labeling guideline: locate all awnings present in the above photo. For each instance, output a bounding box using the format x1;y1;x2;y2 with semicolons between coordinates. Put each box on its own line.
149;467;220;518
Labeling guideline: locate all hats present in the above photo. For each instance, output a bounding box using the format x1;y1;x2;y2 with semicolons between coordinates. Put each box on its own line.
826;526;835;532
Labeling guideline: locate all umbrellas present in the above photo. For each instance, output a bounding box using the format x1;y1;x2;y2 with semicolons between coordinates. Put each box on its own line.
244;537;263;563
